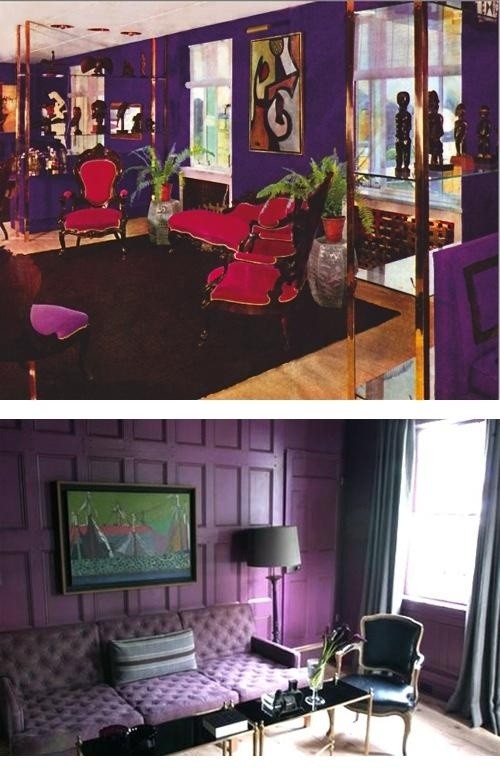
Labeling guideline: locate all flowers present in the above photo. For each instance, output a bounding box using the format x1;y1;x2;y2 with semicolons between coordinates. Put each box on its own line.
313;620;368;683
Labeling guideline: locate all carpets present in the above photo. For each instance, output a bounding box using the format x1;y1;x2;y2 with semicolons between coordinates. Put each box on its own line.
0;231;401;398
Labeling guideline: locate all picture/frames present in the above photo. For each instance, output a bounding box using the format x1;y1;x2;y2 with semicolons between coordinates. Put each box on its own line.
53;477;198;596
249;31;305;156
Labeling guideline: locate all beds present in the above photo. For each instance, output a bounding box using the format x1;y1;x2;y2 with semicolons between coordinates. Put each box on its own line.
235;671;374;755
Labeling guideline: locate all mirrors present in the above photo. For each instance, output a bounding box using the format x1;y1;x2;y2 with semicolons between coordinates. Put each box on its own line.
108;102;145;142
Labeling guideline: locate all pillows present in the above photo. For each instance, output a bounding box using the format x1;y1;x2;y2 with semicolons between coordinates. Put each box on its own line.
109;629;198;685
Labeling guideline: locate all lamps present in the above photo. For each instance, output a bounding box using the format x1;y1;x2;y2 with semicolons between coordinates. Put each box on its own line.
240;523;302;643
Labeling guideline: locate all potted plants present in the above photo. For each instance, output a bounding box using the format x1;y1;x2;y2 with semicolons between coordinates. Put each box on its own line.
123;143;218;203
252;146;377;242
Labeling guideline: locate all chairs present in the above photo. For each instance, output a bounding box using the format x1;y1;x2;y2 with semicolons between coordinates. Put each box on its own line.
0;245;93;399
168;172;334;353
326;612;426;756
0;167;15;241
59;143;131;260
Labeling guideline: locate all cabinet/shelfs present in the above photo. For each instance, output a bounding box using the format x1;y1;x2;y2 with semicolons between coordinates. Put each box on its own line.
17;22;168;245
345;3;499;398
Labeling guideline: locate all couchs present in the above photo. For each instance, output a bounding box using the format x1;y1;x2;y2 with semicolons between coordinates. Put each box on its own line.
0;602;302;754
